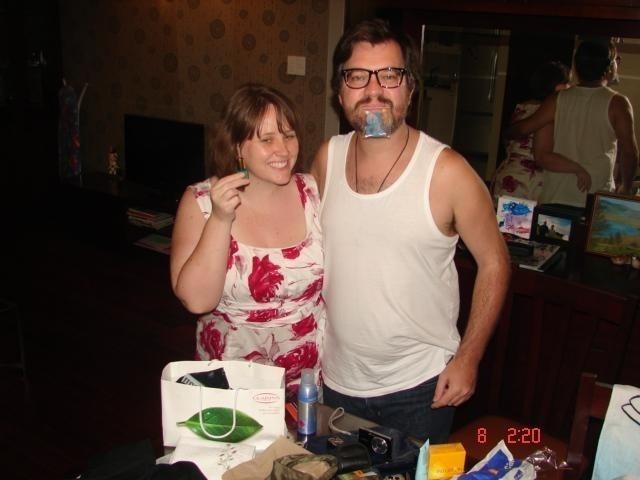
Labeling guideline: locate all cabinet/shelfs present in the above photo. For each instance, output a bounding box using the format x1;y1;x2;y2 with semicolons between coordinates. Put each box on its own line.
416;24;512;182
47;171;180;252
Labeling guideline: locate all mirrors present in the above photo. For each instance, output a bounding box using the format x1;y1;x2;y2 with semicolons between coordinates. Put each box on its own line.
377;7;640;206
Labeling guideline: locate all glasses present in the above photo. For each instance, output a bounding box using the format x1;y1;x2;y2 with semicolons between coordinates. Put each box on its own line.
616;56;621;66
341;66;410;89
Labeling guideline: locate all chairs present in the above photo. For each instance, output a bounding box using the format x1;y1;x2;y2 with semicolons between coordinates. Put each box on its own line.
447;266;635;480
563;373;613;480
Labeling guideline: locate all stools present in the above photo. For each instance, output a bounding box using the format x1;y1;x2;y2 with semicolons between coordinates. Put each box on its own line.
0;298;26;380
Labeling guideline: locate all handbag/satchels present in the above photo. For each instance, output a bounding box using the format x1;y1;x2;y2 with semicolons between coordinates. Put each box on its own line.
159;358;287;453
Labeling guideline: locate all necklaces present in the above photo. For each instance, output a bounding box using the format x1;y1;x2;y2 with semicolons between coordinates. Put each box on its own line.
354;128;411;194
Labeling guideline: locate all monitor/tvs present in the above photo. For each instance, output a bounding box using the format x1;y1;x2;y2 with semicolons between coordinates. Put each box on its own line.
124;114;210;216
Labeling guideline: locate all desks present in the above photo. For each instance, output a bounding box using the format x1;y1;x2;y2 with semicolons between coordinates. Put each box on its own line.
507;243;640;442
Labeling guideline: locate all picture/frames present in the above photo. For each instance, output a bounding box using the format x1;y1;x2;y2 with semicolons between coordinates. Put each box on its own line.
584;192;640;258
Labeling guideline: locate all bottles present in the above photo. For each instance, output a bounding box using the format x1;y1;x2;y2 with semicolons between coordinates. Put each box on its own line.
297;367;317;435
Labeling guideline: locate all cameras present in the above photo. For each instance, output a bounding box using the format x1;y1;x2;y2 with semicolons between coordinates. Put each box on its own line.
358;424;403;462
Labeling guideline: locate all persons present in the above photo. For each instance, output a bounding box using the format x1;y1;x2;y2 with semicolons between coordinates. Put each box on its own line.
502;32;638;219
490;62;592;209
170;84;324;414
310;23;515;447
